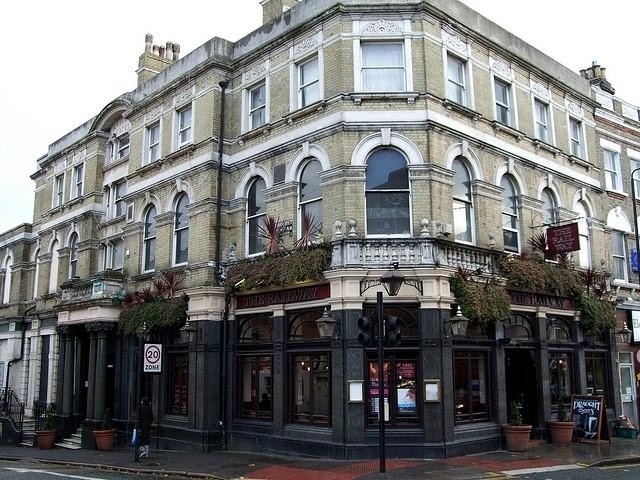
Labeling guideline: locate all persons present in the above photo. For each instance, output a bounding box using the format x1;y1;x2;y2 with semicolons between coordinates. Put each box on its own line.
135;397;153;460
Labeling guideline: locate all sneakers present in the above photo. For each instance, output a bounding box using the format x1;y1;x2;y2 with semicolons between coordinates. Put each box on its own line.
138;452;146;459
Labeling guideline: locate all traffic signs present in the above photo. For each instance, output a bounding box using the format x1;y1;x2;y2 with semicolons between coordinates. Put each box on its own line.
144;343;163;372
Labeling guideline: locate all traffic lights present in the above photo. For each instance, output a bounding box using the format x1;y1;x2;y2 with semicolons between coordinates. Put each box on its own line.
357;314;403;347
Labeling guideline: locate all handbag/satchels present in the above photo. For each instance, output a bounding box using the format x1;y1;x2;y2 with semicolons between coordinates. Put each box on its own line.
132;428;137;446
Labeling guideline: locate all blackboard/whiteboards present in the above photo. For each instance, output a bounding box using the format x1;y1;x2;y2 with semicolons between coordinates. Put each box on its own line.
569;394;603;444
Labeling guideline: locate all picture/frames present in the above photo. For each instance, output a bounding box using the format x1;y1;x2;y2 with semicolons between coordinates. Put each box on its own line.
346;378;366;405
423;378;441;403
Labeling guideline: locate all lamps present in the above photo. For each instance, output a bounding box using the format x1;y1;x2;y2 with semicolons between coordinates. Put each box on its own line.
179;318;202;344
381;262;405;296
445;305;470;339
315;307;340;337
144;327;157;343
612;321;633;344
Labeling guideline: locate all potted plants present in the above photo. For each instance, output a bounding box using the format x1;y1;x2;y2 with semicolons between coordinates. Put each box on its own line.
548;396;576;445
501;400;533;452
92;408;116;451
35;407;57;450
